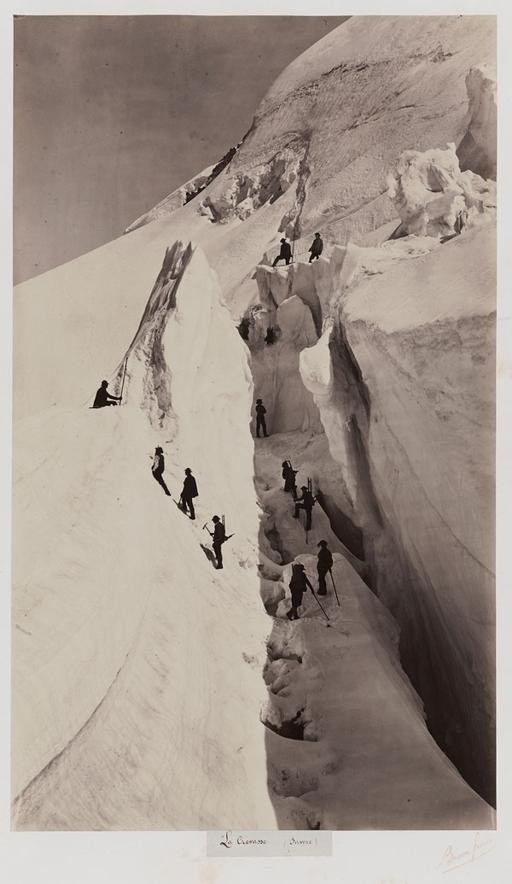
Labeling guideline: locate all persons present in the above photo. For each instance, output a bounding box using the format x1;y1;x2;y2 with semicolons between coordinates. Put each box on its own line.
266;325;275;344
238;318;250;339
255;397;269;438
180;467;198;520
307;230;323;261
152;446;165;478
281;460;298;500
210;514;226;569
286;562;307;621
93;379;122;407
293;485;315;530
316;538;333;596
271;236;292;266
